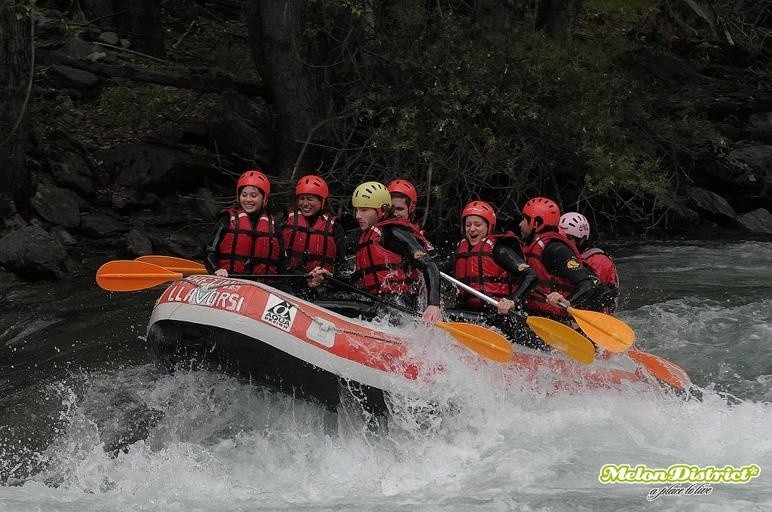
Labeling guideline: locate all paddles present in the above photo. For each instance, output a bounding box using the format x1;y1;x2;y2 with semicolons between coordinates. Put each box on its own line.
323;273;513;363
96;256;321;292
439;271;635;363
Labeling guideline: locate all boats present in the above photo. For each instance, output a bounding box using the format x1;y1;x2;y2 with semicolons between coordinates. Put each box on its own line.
143;273;698;432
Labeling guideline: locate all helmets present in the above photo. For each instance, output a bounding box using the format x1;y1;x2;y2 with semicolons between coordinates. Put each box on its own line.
386;176;419;215
558;211;591;242
521;195;562;234
235;170;271;209
350;180;393;211
294;174;328;211
459;200;496;236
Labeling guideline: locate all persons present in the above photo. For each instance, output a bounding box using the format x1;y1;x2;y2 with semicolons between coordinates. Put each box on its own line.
202;173;619;329
